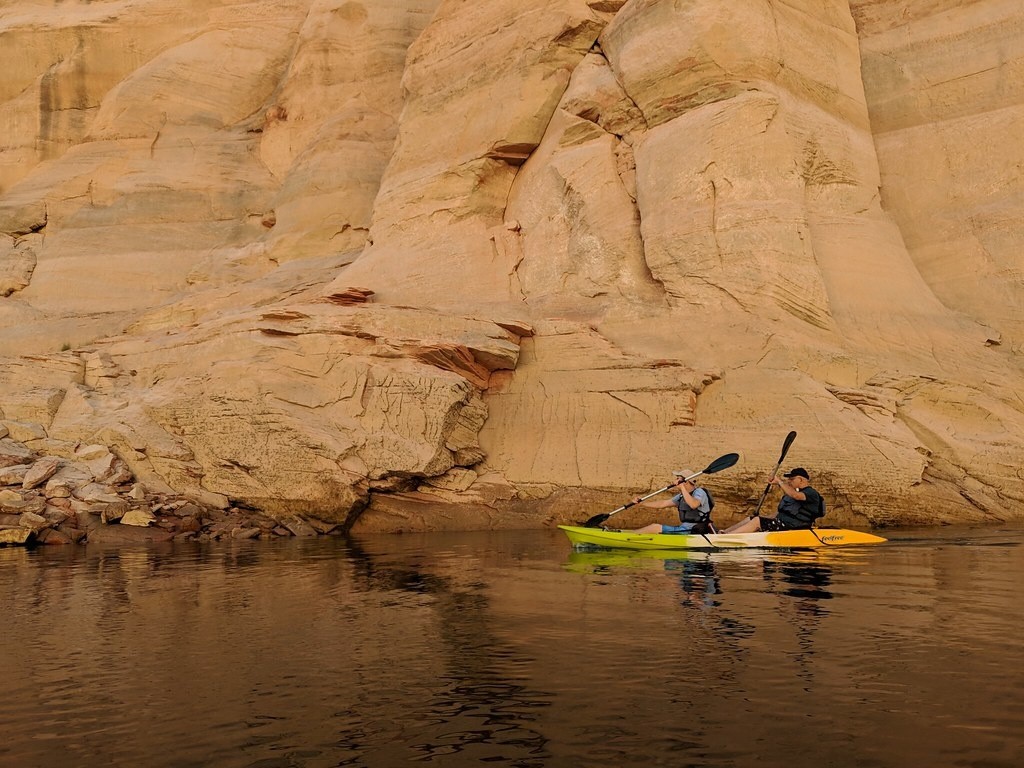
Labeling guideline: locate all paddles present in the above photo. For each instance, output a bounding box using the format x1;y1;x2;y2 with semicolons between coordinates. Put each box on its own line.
748;430;799;520
582;451;740;528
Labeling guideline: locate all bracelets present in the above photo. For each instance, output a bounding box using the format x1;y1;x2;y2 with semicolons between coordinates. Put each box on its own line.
778;481;783;486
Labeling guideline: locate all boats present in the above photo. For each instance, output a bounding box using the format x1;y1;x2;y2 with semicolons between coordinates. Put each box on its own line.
558;522;890;548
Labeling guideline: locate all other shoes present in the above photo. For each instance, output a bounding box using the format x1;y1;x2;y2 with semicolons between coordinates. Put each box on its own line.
709;523;723;535
604;525;622;532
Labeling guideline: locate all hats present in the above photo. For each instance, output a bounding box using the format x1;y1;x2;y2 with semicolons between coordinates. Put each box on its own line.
783;468;809;479
673;470;698;485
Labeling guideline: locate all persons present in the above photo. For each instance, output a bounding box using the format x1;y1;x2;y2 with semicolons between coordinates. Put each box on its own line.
604;469;711;535
708;468;819;534
